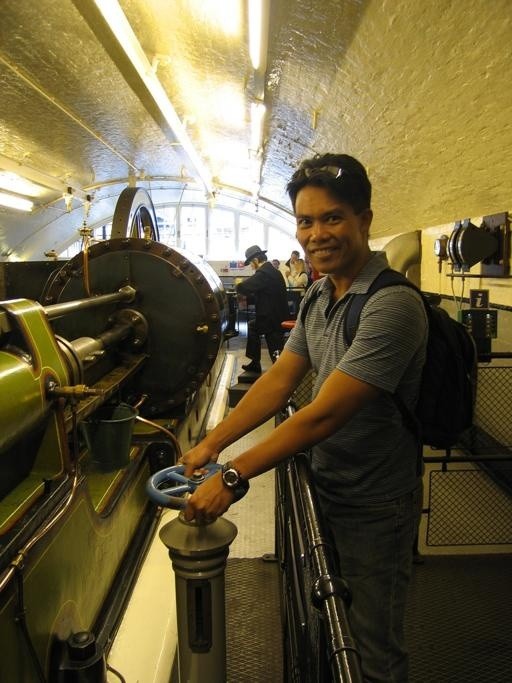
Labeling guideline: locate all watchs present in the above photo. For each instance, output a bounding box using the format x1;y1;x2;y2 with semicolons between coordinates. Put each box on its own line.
222;463;245;495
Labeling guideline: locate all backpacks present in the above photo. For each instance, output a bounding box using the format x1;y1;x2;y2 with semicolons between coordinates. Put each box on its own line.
343;268;477;450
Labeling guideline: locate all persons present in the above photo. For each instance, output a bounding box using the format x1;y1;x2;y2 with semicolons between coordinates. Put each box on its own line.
175;152;430;683
272;251;319;315
234;246;290;372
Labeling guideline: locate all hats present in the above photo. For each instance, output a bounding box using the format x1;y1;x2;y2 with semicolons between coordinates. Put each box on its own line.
243;245;267;266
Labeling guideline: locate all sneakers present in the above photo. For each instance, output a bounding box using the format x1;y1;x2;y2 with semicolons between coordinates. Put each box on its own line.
242;362;262;374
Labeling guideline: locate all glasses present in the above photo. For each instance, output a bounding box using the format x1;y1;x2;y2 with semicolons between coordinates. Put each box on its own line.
292;166;349;182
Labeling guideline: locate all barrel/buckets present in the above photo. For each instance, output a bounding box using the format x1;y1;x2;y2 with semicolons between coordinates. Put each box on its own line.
80;402;139;468
